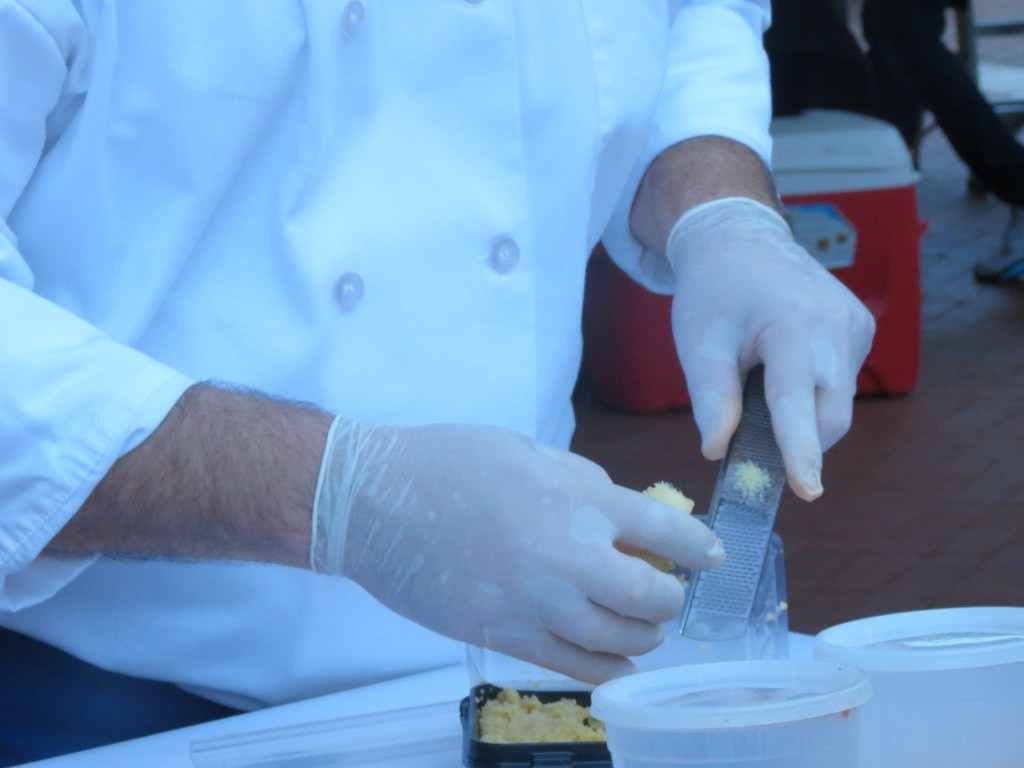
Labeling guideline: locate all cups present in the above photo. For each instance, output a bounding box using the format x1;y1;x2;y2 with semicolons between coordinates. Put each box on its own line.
589;658;876;768
815;606;1024;768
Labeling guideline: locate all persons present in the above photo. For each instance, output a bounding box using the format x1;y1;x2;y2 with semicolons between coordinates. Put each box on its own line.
0;0;878;768
858;0;1024;283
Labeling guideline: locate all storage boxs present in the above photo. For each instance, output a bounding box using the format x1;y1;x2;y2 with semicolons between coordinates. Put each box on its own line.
581;108;925;414
459;514;790;768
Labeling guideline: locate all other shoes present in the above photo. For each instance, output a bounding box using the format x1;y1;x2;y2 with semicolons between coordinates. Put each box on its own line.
973;204;1024;283
